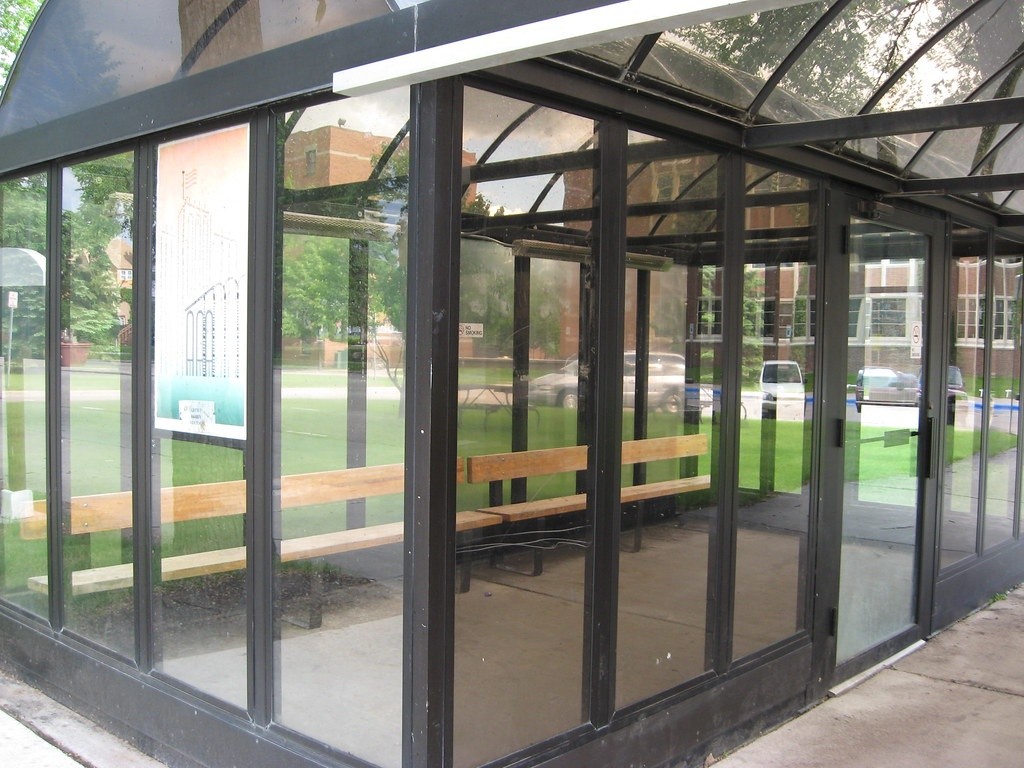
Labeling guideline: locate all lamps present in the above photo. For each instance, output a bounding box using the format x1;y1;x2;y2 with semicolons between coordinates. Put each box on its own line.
512;239;672;272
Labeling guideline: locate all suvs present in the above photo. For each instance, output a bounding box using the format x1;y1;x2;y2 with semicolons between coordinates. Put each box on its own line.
528;349;686;413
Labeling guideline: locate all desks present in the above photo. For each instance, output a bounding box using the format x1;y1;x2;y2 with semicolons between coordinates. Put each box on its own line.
459;383;538;431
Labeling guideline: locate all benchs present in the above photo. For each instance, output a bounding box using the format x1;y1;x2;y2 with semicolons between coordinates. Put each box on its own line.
467;434;710;577
19;455;503;654
282;210;402;244
856;385;919;413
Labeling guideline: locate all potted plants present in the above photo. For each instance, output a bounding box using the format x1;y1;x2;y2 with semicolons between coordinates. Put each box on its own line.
61;328;92;368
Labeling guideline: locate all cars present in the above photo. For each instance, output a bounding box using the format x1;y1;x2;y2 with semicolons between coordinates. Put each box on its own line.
758;359;809;403
854;365;968;405
